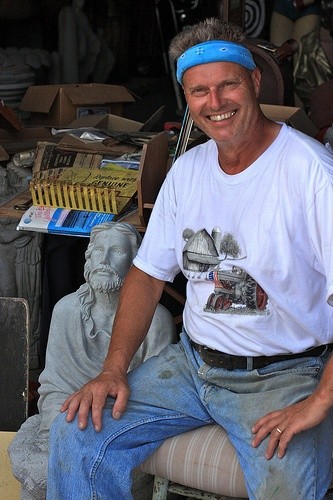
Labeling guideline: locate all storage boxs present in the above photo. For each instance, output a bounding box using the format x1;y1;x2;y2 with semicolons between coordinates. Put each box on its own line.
66;104;166;133
259;104;320;138
19;83;142;128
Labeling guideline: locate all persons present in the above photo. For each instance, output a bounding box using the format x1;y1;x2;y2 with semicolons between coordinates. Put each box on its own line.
47;18;333;500
7;221;174;500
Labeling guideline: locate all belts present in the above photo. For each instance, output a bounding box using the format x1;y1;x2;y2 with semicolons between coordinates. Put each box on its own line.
182;329;326;371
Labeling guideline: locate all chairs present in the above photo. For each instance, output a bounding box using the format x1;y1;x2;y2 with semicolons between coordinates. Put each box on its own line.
140;421;250;500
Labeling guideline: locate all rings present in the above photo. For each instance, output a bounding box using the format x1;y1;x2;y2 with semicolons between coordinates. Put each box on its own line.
276;427;283;434
